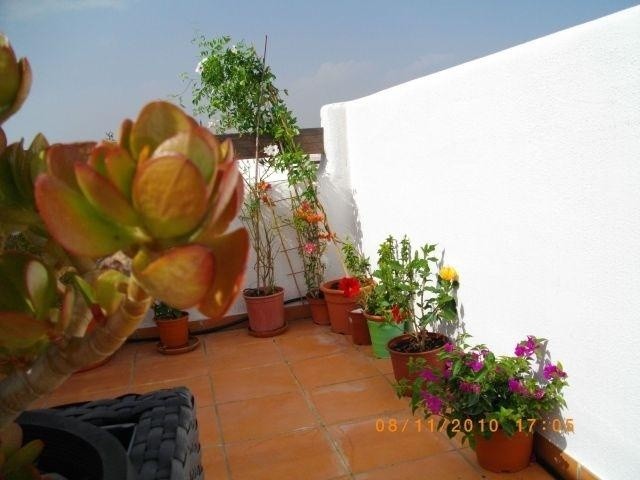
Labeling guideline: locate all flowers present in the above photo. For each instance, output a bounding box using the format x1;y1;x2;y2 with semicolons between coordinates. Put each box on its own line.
414;339;569;432
389;245;458;340
166;34;332;299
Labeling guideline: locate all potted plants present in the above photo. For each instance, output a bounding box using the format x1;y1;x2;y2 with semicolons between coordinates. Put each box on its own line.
1;36;253;480
150;298;201;356
339;237;407;360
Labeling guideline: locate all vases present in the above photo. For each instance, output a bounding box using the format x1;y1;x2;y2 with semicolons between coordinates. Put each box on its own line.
13;410;141;479
244;285;289;337
385;331;453;400
306;291;331;326
320;276;374;335
474;419;538;472
